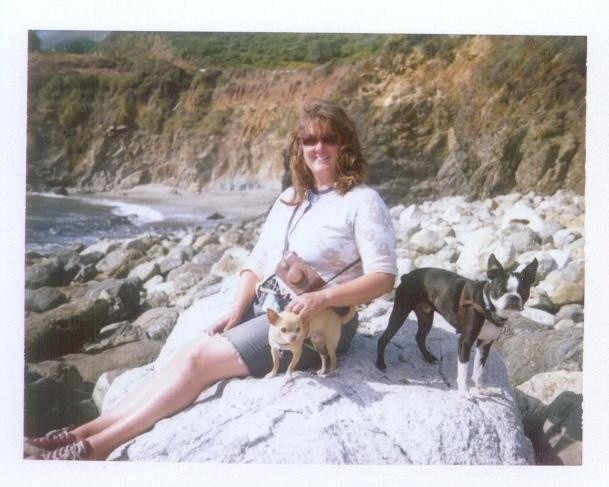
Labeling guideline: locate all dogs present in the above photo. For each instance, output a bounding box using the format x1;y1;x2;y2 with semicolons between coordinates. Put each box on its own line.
263;306;357;384
375;254;538;401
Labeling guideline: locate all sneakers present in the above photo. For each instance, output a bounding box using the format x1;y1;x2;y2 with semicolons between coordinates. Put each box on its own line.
24;439;90;460
24;423;77;455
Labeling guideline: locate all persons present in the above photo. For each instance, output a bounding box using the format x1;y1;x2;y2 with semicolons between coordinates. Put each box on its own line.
26;96;401;461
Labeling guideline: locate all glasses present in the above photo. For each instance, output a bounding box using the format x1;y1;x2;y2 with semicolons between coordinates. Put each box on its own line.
300;131;341;146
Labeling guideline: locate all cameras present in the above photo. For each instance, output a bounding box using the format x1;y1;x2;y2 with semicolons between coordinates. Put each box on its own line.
275;251;324;296
